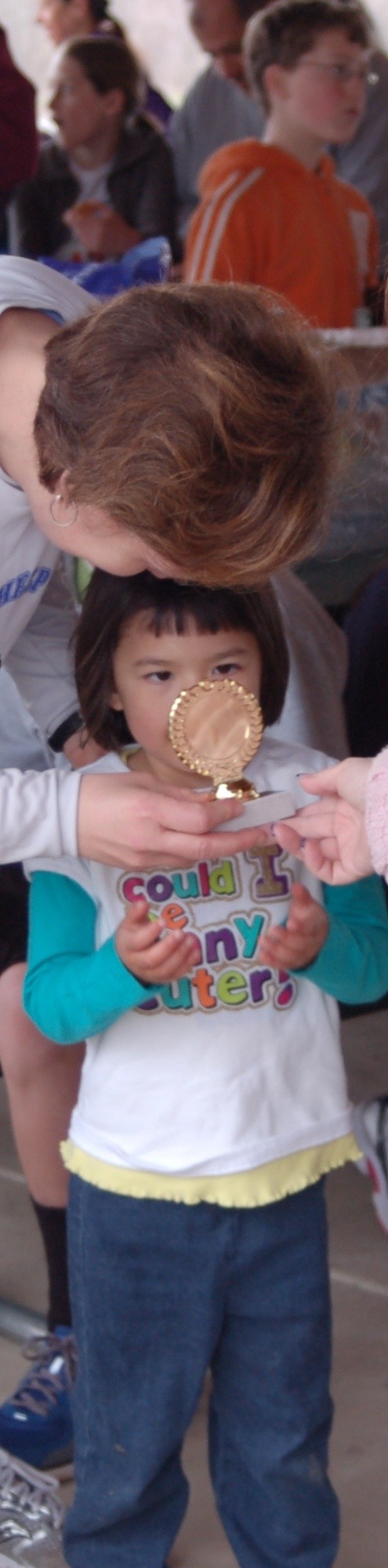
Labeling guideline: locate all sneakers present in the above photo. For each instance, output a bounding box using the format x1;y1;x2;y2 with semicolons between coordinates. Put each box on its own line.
0;1324;78;1488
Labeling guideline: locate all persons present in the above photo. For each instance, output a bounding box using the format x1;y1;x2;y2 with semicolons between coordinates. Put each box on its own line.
0;0;388;1482
36;563;388;1568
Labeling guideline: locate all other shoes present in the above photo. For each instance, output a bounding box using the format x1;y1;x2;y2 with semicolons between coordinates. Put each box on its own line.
350;1098;388;1236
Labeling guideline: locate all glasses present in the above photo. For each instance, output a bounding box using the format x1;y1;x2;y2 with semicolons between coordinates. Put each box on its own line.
297;58;380;89
